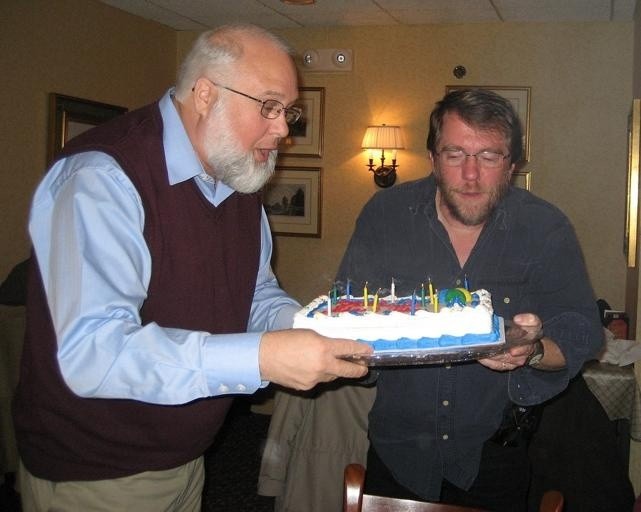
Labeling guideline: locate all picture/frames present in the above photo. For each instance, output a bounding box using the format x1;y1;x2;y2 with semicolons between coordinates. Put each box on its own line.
277;88;323;157
51;92;130;160
443;86;641;269
258;165;324;237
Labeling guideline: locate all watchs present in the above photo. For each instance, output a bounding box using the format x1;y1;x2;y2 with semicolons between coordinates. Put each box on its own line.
524;339;544;367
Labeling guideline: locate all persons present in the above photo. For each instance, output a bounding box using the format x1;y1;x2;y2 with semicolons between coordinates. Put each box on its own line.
11;23;374;512
330;88;604;512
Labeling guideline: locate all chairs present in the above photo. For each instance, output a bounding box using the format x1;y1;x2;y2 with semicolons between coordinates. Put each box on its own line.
344;464;565;511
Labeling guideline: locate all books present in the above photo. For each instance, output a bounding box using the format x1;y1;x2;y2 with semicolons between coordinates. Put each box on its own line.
603;309;628;340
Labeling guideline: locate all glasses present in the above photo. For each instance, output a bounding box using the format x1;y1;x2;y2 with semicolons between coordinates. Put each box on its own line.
191;80;302;125
434;147;510;168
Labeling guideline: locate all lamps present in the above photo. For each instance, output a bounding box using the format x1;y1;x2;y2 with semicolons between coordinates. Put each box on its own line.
361;124;407;190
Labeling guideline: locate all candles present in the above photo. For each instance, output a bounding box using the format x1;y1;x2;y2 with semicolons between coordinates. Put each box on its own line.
324;274;471;316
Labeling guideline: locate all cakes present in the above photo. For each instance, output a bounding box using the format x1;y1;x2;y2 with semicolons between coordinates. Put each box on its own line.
294;289;499;351
